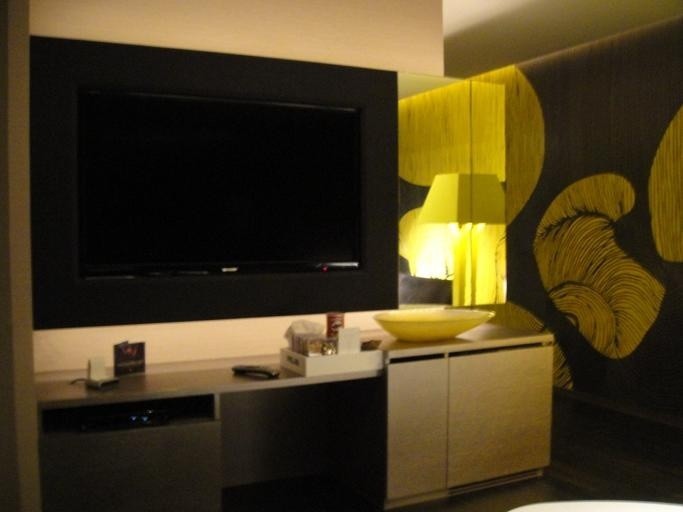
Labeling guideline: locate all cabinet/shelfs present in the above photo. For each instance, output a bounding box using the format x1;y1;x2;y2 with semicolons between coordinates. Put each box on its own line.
33;353;385;511
384;344;557;512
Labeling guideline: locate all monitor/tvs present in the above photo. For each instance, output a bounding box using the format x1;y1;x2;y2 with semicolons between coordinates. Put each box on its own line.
76;87;366;285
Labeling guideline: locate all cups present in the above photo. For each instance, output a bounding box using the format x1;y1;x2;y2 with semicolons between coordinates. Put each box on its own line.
337;325;361;355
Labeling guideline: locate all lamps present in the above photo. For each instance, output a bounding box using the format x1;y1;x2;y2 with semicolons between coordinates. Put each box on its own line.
472;173;504;224
413;171;473;226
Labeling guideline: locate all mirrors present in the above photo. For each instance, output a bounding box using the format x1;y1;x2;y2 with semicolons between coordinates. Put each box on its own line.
400;75;472;307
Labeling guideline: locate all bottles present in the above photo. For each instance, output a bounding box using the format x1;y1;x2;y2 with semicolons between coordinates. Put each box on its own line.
325;311;344;339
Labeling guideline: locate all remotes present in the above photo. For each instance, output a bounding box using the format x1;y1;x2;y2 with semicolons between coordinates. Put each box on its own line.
232;365;278;380
